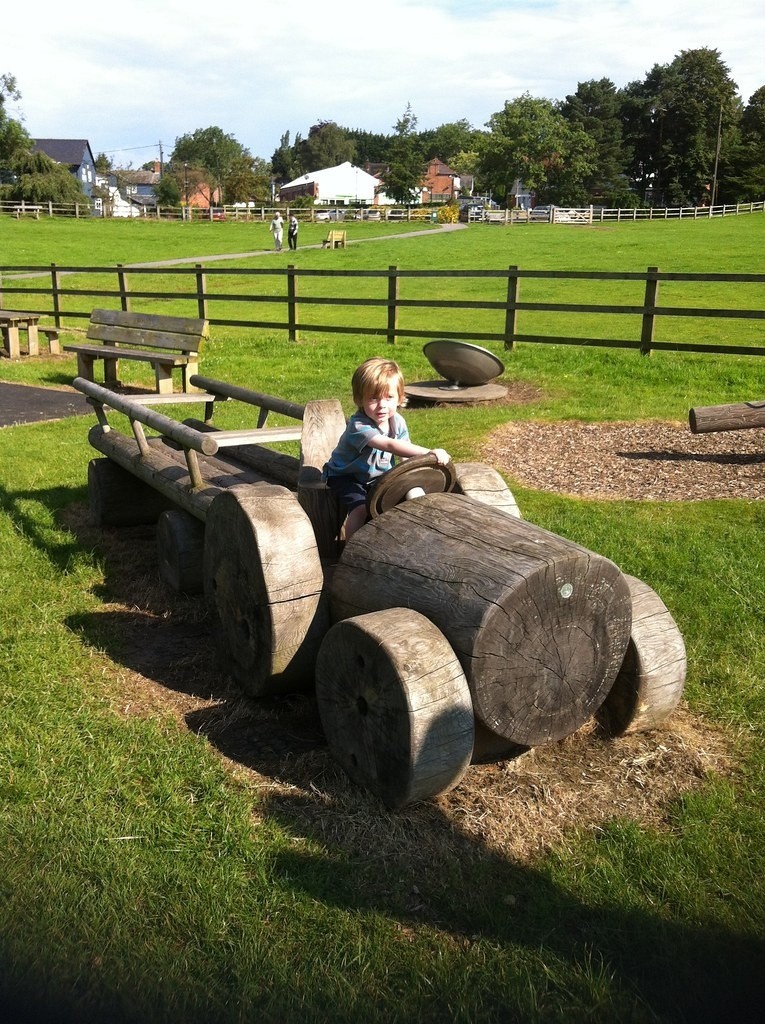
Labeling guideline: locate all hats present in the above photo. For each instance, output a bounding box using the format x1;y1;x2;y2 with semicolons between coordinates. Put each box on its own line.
276;212;281;216
288;213;294;216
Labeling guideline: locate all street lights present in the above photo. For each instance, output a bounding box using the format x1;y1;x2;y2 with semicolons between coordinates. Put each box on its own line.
184;161;188;207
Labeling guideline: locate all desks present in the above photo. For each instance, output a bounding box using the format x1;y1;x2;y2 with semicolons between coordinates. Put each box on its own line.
0;311;49;359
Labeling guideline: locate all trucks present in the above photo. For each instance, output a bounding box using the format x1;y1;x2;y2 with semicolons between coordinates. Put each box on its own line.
70;372;688;811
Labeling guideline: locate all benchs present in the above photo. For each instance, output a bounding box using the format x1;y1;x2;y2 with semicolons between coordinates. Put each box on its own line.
65;308;210;395
322;230;347;250
0;324;61;354
13;205;43;220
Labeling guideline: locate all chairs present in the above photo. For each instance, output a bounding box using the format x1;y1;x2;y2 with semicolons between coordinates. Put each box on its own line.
298;399;348;559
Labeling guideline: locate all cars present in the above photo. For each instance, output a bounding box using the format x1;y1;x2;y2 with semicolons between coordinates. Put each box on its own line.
531;206;550;220
315;209;406;222
459;203;489;222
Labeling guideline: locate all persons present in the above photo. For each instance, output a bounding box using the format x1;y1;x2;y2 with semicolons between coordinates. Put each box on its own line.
323;355;454;548
288;213;298;251
269;212;284;252
